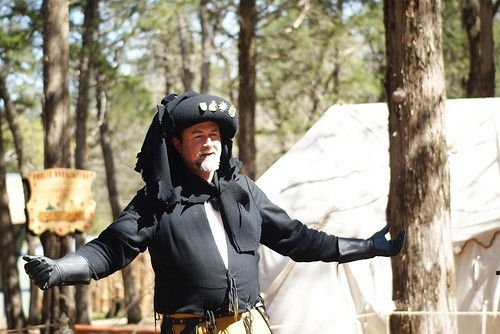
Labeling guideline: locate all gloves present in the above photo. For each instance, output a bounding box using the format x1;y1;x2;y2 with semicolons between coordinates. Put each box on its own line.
23;251;92;290
337;220;406;264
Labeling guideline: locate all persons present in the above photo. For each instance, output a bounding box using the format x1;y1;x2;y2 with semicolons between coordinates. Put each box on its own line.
21;91;405;333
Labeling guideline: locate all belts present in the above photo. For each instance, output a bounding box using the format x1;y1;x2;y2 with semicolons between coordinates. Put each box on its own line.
162;314;225;324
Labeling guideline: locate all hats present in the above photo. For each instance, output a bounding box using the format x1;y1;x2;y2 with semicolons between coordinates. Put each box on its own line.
133;91;242;210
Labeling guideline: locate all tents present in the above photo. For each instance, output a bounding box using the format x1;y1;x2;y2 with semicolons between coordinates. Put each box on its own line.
255;95;499;334
